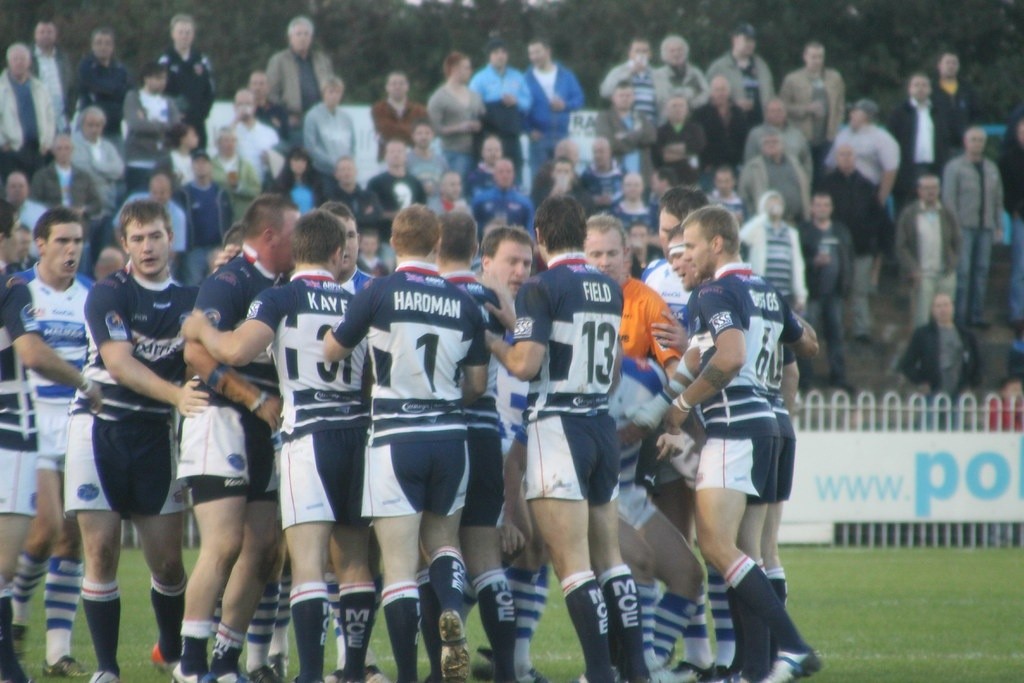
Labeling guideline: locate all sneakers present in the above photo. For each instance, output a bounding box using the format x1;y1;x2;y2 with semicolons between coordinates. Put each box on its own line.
267;654;287;677
522;668;549;683
366;665;391;683
715;671;752;683
42;655;89;678
670;661;715;682
9;623;27;661
171;663;248;683
250;666;282;683
325;669;343;682
88;671;121;683
152;641;181;672
574;669;651;683
477;647;493;661
761;648;822;683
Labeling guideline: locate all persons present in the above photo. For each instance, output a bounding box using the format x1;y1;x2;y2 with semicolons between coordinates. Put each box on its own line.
0;14;1023;683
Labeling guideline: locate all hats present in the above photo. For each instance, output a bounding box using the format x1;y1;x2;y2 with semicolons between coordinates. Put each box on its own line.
736;23;758;39
846;97;878;117
486;39;507;52
192;149;211;163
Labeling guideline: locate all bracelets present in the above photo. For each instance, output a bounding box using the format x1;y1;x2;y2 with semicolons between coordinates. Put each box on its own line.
672;395;692;412
79;376;92;394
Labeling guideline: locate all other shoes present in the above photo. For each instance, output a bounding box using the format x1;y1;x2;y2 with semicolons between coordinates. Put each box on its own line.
831;377;857;395
972;320;991;331
858;334;870;343
439;609;470;682
